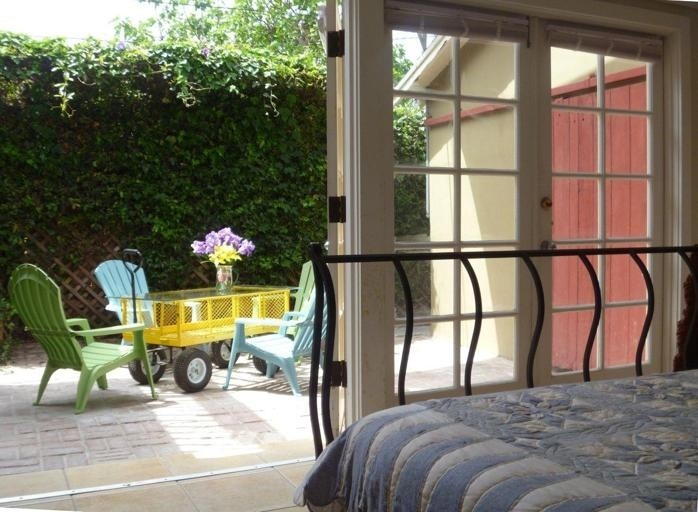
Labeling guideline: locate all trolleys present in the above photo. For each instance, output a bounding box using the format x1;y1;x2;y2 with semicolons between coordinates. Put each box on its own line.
120;286;290;392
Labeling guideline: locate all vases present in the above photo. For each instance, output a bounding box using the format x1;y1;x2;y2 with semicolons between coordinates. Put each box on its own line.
216;265;233;295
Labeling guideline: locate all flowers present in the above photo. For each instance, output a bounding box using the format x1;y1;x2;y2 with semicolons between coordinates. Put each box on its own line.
191;227;256;293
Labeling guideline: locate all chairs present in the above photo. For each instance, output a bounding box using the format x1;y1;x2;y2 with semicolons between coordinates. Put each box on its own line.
7;260;203;415
222;258;328;397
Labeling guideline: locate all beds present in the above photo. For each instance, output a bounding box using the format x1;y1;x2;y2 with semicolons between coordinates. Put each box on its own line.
304;242;698;511
